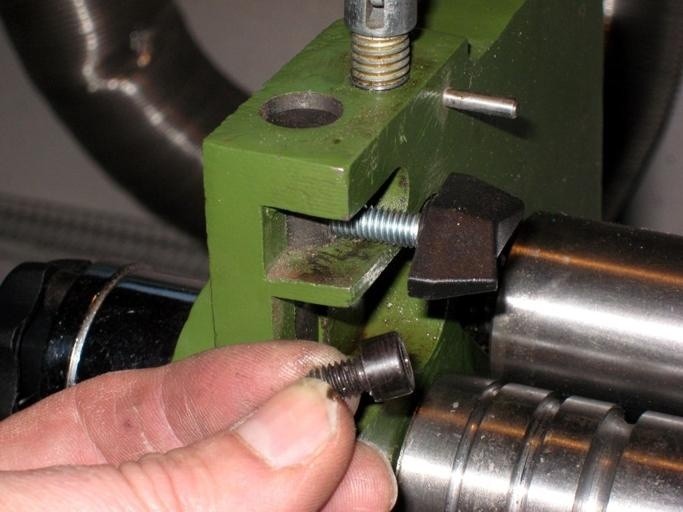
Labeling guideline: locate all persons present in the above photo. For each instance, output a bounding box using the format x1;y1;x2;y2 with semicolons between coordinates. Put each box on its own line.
0;340;399;512
